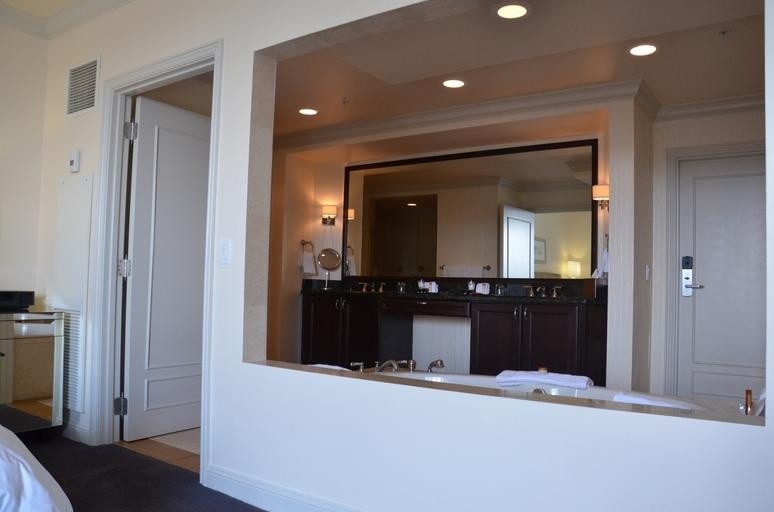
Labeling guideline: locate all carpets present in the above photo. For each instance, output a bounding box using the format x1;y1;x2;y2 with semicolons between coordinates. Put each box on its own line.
0;405;265;512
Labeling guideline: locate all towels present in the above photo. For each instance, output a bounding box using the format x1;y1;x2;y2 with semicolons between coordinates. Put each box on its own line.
443;263;484;278
303;252;315;275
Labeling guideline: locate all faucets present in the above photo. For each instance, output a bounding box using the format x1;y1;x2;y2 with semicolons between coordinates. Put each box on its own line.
426;359;444;373
376;359;400;372
535;284;549;298
368;280;377;291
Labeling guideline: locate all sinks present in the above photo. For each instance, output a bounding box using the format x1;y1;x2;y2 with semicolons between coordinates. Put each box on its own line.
385;371;703;413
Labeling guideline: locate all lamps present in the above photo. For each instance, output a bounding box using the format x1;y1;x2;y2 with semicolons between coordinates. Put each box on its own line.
566;257;581;279
348;209;355;219
592;185;610;209
322;206;337;225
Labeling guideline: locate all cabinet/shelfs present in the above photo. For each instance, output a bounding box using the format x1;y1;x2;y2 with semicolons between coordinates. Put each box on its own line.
301;296;382;368
387;302;471;319
475;302;581;379
0;312;65;434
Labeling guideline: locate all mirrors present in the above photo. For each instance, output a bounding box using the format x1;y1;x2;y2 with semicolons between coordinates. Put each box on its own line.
341;137;598;287
317;247;341;290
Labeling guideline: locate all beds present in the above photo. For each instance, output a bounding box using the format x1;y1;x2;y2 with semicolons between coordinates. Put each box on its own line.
0;424;74;512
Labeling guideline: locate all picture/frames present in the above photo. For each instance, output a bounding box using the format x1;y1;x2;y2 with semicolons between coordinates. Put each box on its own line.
534;237;547;264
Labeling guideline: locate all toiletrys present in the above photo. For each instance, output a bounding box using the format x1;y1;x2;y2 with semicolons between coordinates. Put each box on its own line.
494;277;506;296
